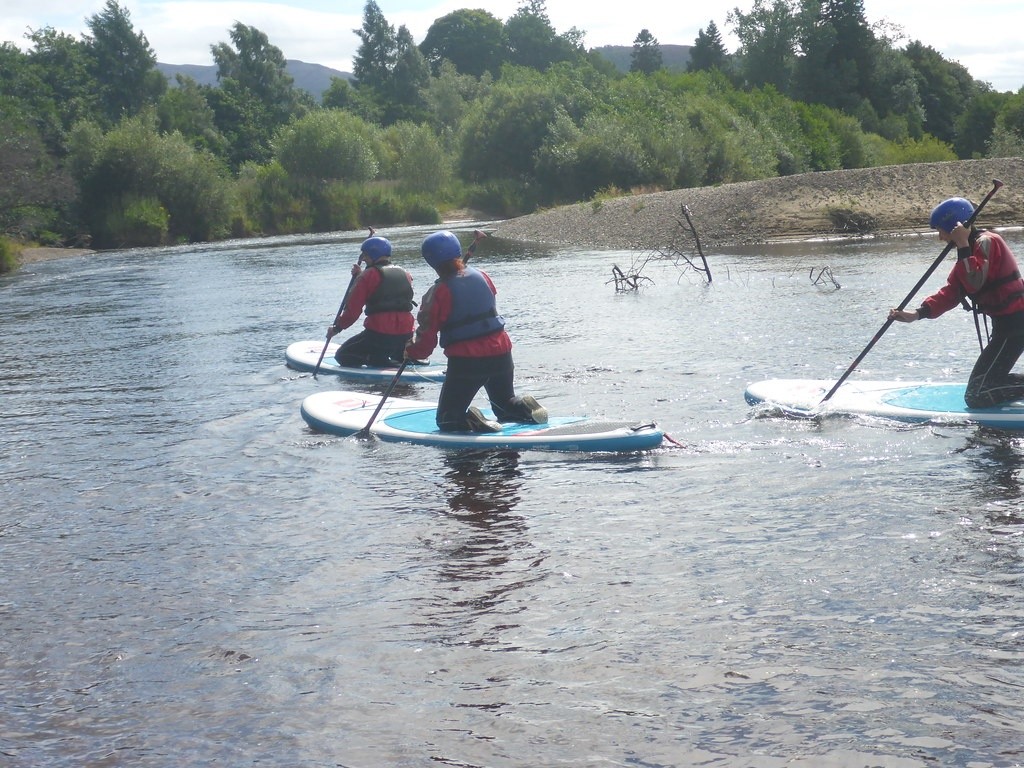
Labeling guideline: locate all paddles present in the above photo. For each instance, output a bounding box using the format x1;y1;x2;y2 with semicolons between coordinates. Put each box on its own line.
818;178;1005;409
346;229;490;440
312;226;377;376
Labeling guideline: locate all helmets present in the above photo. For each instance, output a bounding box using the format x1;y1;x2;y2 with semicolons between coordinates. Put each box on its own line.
422;230;462;267
930;198;975;234
361;237;392;261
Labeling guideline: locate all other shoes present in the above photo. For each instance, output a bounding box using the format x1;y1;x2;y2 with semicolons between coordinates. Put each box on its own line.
522;396;549;424
414;358;430;365
467;406;503;433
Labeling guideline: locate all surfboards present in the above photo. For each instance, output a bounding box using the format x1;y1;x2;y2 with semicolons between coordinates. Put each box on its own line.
285;340;447;383
743;377;1024;431
300;389;664;453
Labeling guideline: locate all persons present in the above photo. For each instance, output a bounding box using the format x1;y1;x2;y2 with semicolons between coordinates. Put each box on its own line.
324;237;431;368
886;197;1024;409
402;230;549;430
